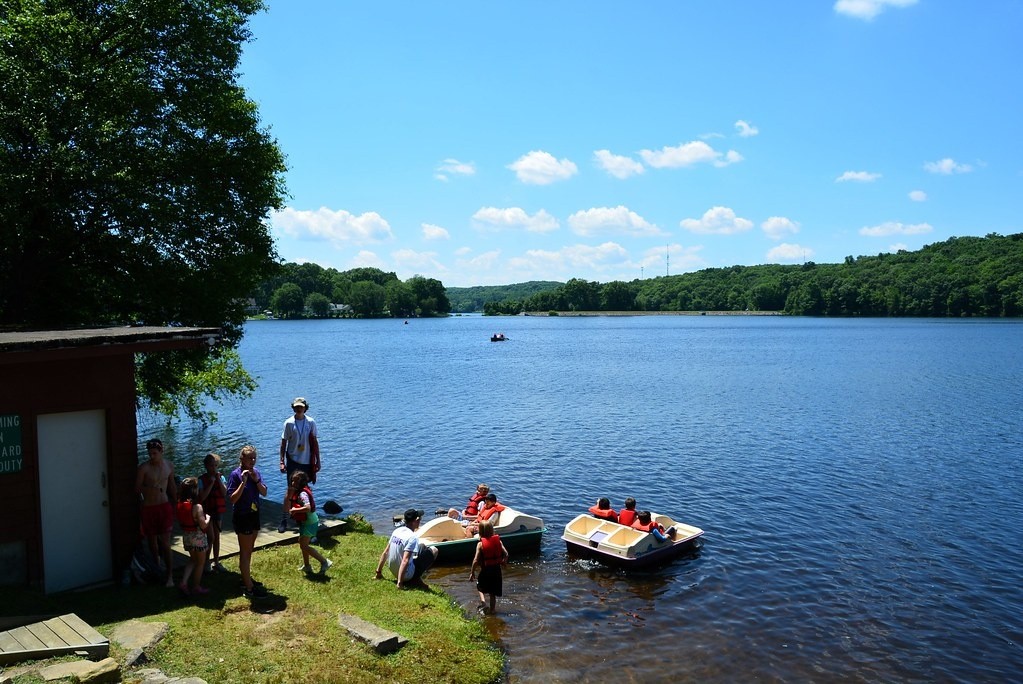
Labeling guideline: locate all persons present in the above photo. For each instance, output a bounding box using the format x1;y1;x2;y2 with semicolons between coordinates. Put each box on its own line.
135;438;177;589
468;519;508;614
465;494;498;538
631;510;677;541
588;497;618;523
287;470;332;573
197;453;226;574
619;497;639;526
277;397;321;532
227;444;268;599
447;483;489;527
371;508;438;591
176;476;211;596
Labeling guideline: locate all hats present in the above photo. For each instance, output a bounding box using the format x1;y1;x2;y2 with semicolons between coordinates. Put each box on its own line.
404;509;423;522
292;397;306;408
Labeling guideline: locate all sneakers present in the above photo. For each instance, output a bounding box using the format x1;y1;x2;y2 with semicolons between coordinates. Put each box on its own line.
243;590;268;599
240;578;262;587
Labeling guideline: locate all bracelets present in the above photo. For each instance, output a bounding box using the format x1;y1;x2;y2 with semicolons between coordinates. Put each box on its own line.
375;570;380;573
254;479;260;484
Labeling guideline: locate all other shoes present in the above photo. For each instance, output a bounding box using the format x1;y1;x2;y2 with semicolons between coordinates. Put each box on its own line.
319;559;332;575
194;587;207;592
298;565;311;571
166;580;175;587
178;582;188;590
278;520;287;532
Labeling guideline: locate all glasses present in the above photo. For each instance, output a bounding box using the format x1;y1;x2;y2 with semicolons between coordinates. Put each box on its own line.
146;442;161;448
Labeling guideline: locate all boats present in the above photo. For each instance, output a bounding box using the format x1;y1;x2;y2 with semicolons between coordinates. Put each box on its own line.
561;511;706;576
490;333;505;342
392;503;548;562
